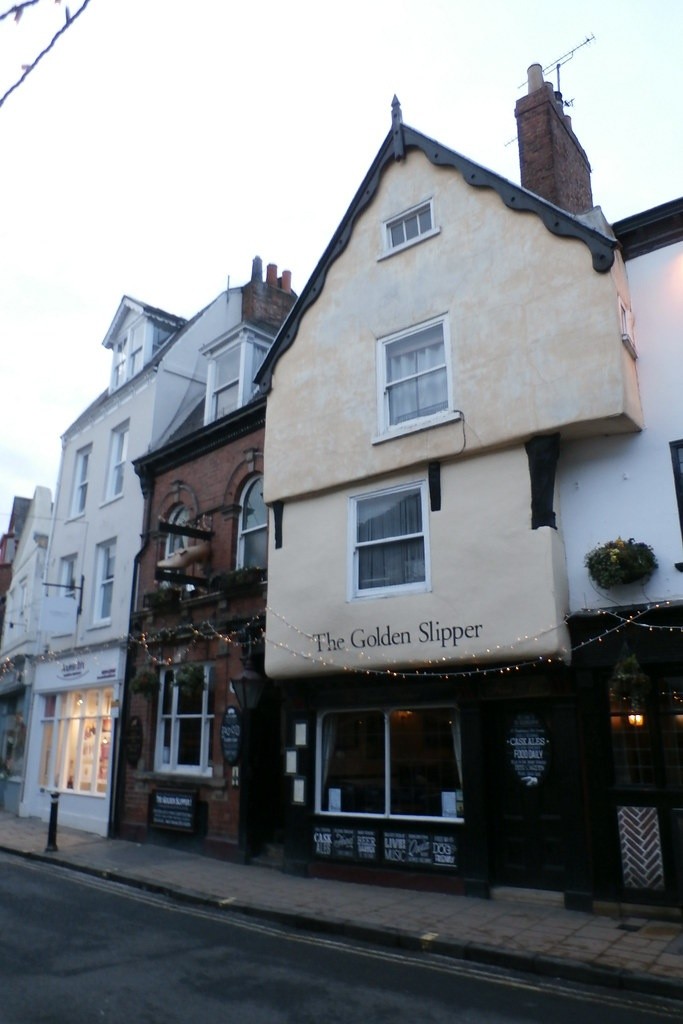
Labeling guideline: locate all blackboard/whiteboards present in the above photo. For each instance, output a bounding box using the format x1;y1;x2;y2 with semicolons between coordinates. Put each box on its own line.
147;788;197;831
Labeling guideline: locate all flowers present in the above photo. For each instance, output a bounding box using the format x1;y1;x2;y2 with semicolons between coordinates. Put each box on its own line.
583;536;658;590
